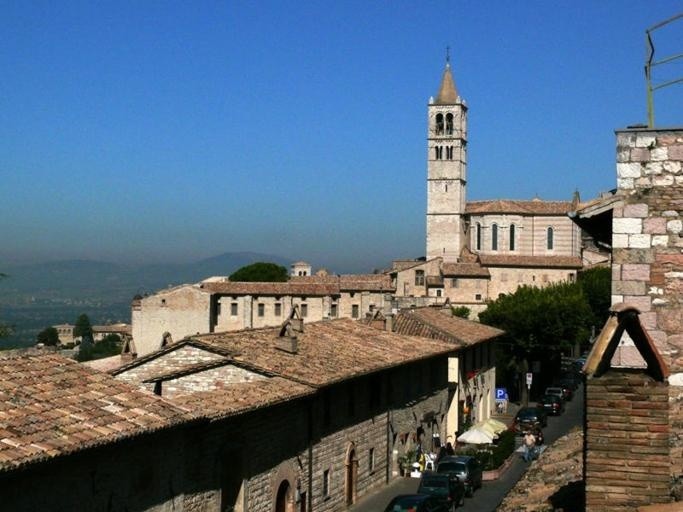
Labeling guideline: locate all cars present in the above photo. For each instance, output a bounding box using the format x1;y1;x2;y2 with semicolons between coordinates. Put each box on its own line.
513;357;587;446
384;495;449;512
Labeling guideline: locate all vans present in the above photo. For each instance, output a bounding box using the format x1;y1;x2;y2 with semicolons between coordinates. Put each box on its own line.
435;456;482;497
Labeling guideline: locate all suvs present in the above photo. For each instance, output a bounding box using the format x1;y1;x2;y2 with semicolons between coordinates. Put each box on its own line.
418;472;464;512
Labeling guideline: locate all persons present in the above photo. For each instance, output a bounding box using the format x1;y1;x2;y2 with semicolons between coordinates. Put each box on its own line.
497;402;503;414
432;442;456;464
523;428;544;462
